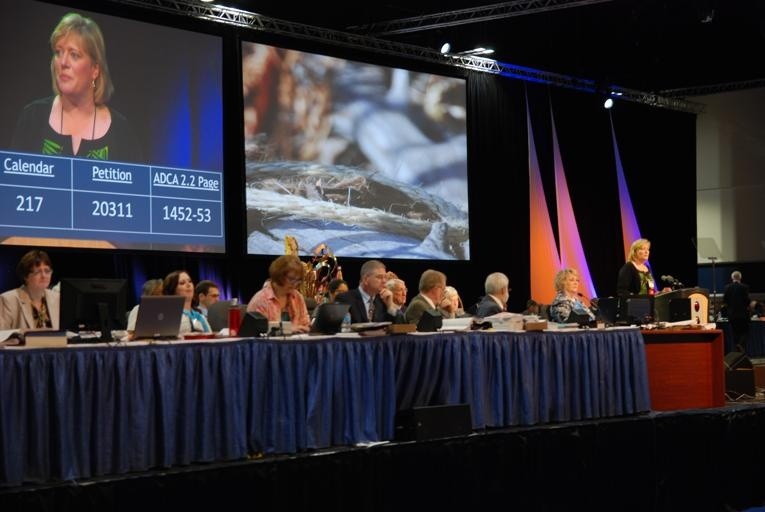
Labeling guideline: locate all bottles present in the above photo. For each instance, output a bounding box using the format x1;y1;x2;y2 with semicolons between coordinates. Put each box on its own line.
549;290;570;323
341;311;352;333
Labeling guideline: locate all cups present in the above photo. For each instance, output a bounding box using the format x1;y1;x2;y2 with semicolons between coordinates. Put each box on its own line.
227;305;242;337
596;320;606;329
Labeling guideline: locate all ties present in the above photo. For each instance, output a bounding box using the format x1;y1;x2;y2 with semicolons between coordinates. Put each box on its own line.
368;296;375;322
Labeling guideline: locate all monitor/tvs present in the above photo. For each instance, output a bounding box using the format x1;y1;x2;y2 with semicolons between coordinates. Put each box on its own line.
59;278;128;344
622;294;655;325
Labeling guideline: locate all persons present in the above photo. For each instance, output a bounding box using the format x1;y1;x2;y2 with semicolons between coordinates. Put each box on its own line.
0;250;61;333
549;268;597;330
722;270;751;353
521;299;550;318
11;15;141;165
616;239;672;324
125;255;510;343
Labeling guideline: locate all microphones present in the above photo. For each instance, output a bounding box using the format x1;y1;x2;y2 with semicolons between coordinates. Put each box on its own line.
578;293;597;307
661;274;684;290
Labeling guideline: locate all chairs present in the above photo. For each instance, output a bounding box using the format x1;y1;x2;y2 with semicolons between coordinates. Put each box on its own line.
125;310;130;322
540;304;553;319
305;297;316;314
208;301;246;332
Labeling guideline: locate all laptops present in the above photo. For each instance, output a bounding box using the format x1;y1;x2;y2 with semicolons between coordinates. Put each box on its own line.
127;295;186;341
295;303;350;333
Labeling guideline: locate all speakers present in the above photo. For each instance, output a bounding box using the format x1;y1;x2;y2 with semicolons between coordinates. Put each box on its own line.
393;404;473;438
723;351;756;398
